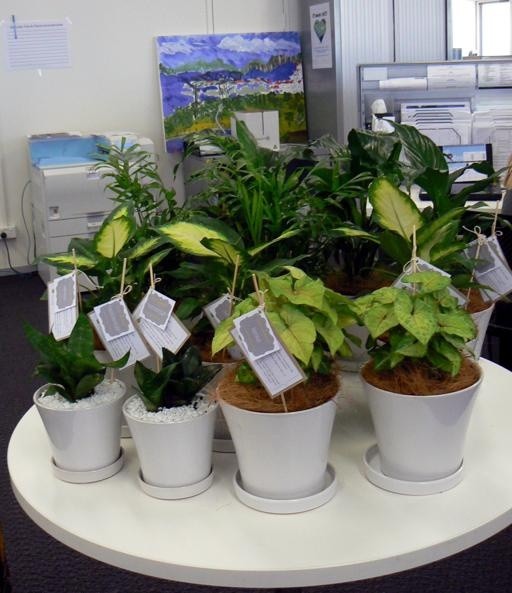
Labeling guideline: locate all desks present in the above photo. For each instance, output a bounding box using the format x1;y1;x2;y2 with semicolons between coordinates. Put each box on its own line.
6;354;512;589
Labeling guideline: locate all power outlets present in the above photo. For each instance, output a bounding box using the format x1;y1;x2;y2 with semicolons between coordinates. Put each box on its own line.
0;225;17;240
